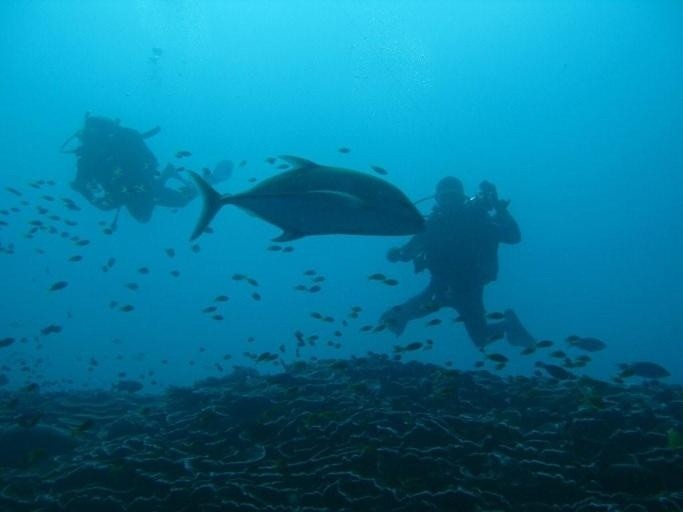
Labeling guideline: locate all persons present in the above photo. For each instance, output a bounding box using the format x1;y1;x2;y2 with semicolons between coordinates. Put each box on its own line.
377;172;538;352
68;112;236;226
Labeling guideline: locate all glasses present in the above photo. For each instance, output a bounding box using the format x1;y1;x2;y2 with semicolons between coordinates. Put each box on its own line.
436;190;460;205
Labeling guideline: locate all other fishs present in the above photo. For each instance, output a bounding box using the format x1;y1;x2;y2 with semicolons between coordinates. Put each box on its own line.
0;144;682;512
